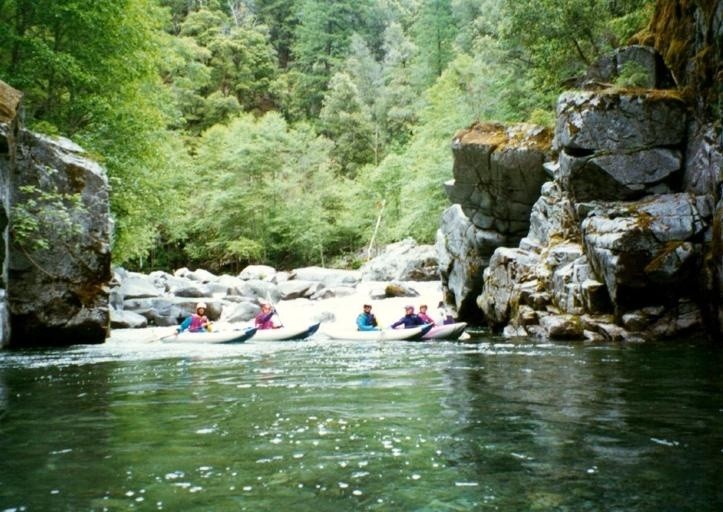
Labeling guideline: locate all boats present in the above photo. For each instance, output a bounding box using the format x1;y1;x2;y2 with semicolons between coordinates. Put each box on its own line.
426;321;467;341
323;321;435;342
250;319;323;343
156;324;256;348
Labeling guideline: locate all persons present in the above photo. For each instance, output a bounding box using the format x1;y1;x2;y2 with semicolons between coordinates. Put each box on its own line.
175;303;212;335
256;304;282;329
391;302;448;329
356;305;381;330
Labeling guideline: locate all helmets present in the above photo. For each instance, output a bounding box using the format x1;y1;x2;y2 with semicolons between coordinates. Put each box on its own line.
261;304;271;312
196;303;206;310
